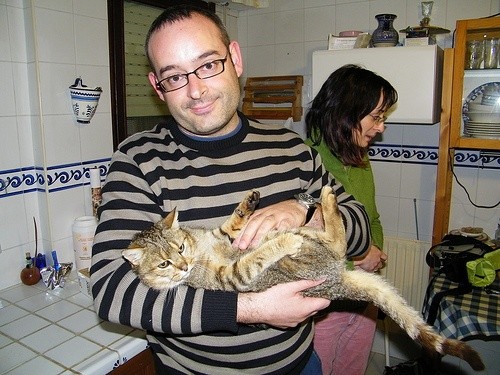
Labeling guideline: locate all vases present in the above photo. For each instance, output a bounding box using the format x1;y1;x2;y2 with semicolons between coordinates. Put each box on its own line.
371;13;399;48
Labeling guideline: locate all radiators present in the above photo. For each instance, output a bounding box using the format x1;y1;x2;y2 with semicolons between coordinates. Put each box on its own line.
375;236;433;318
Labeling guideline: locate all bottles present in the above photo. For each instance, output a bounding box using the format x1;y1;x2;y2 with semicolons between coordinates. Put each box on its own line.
73;216;98;274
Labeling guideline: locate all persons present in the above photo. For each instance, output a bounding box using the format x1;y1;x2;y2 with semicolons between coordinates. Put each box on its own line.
89;9;371;375
302;65;398;375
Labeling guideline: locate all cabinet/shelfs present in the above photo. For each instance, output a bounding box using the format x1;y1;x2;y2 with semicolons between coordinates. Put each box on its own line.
449;18;500;152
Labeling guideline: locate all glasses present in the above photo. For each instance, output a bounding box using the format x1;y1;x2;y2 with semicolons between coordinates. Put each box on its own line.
369;114;388;126
153;49;229;93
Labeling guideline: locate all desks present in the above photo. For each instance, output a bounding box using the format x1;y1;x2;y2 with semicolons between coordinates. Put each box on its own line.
422;266;500;343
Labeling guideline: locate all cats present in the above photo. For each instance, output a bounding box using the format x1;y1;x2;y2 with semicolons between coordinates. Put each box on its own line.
121;186;485;371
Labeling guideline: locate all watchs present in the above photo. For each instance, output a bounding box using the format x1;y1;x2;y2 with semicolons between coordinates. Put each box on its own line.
294;192;318;227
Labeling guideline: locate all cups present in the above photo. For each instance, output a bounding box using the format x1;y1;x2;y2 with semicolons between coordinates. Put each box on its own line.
484;40;498;68
70;78;102;124
466;40;486;69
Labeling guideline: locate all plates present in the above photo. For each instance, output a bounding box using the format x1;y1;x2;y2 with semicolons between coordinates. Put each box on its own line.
463;121;500;139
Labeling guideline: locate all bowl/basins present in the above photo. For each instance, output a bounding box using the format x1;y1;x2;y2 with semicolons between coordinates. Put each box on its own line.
464;112;500;121
467;101;500;111
